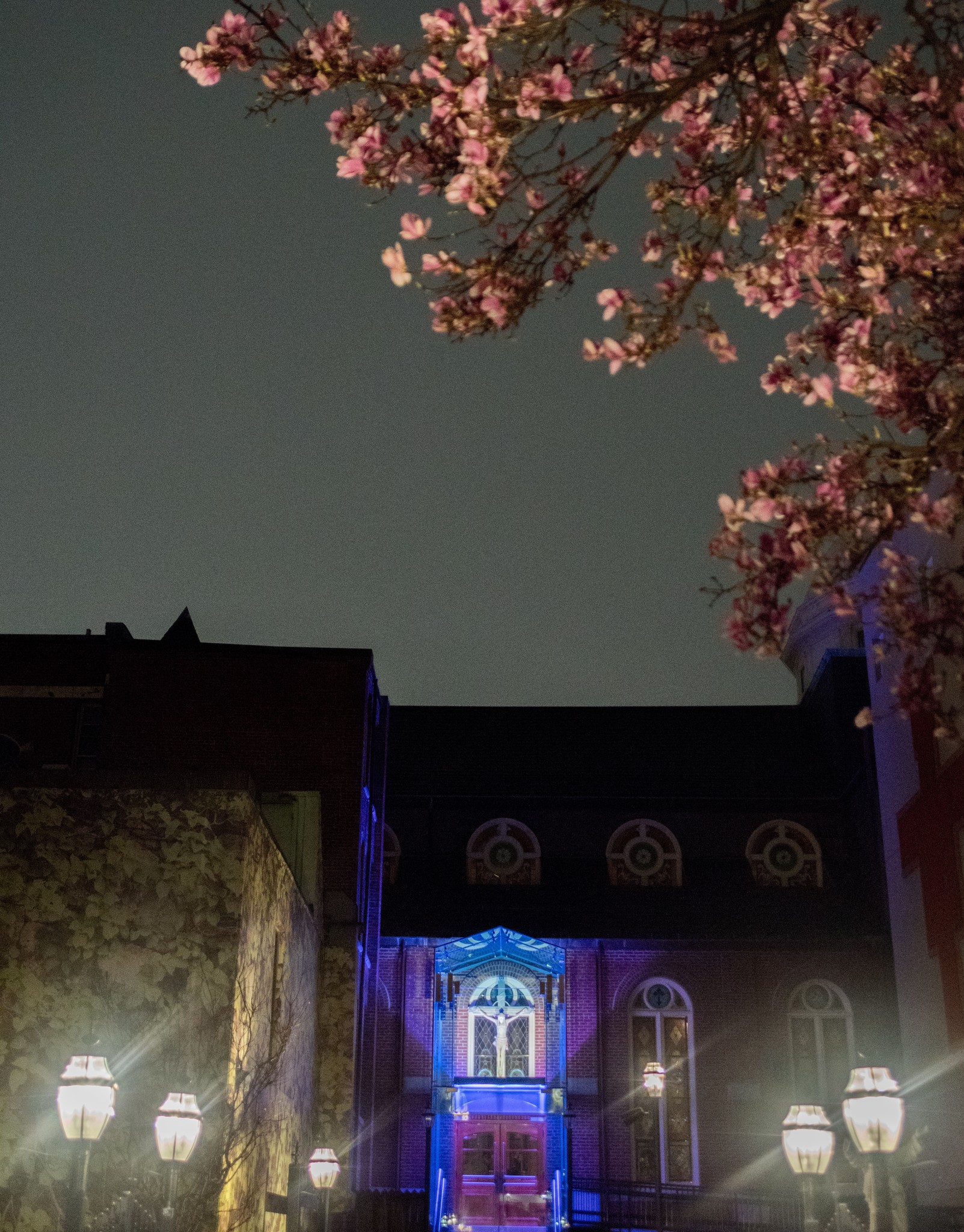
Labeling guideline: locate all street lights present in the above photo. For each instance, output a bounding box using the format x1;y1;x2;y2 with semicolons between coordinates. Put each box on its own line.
839;1067;909;1232
643;1061;670;1231
153;1090;202;1232
307;1146;341;1232
777;1103;839;1232
52;1053;116;1232
